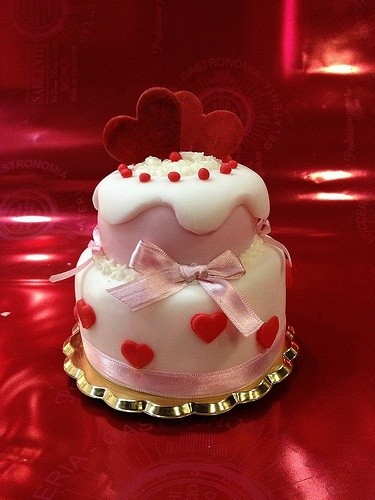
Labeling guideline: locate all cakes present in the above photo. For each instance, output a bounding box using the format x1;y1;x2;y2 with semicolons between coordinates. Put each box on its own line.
71;149;293;400
